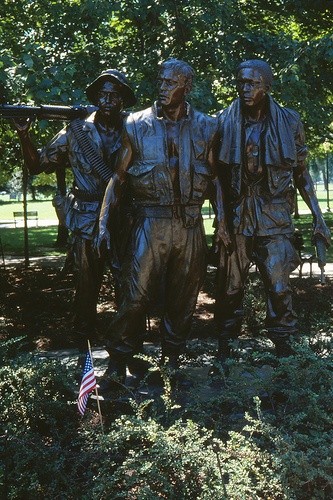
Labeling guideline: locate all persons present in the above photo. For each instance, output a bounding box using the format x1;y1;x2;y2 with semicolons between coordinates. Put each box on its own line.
10;58;333;367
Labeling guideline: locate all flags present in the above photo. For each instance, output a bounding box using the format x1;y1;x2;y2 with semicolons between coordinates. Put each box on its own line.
74;346;100;417
